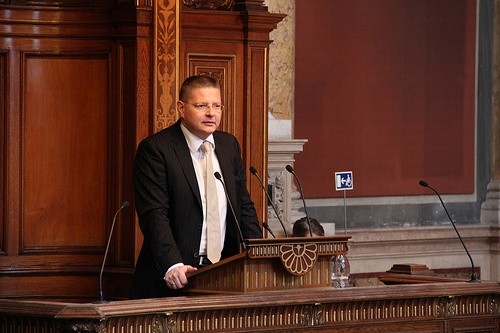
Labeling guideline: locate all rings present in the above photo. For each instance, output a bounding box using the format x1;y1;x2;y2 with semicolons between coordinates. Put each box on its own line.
170;281;176;286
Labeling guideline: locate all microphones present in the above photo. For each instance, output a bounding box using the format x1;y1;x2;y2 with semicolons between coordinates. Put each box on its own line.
262;222;275;238
250;167;287;237
419;180;479;283
214;172;246;251
94;201;130;304
286;165;313;237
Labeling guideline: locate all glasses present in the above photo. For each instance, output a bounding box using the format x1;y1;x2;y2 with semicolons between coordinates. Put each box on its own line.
182;101;223;109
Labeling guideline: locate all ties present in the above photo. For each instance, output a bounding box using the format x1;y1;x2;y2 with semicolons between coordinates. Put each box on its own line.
201;142;222;263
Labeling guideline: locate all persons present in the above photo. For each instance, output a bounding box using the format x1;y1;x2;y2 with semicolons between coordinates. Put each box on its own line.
293;217;325;237
128;75;263;300
